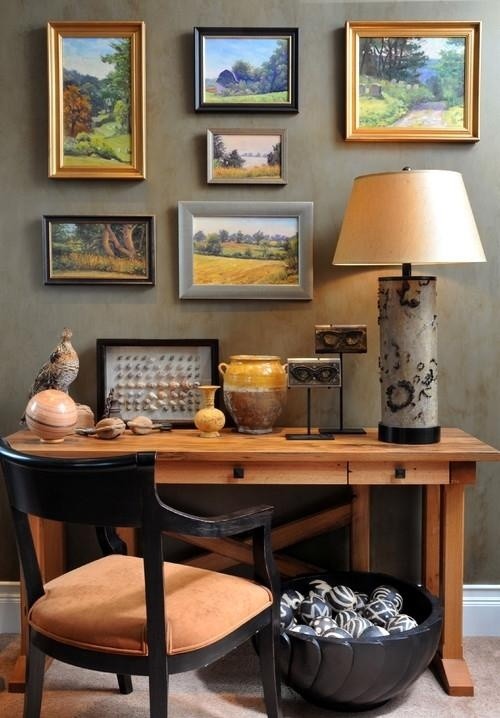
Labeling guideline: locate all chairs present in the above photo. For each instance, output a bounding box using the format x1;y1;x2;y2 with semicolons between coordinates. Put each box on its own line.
0;443;284;718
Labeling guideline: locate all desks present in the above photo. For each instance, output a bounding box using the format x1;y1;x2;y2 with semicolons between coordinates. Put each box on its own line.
1;426;500;697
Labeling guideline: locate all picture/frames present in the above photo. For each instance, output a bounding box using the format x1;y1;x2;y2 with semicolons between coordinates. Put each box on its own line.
42;213;155;286
48;19;146;180
177;201;314;301
194;26;300;114
344;20;482;144
206;127;289;185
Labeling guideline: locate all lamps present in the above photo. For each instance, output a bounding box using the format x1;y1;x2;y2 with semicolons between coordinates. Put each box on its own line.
330;166;487;446
